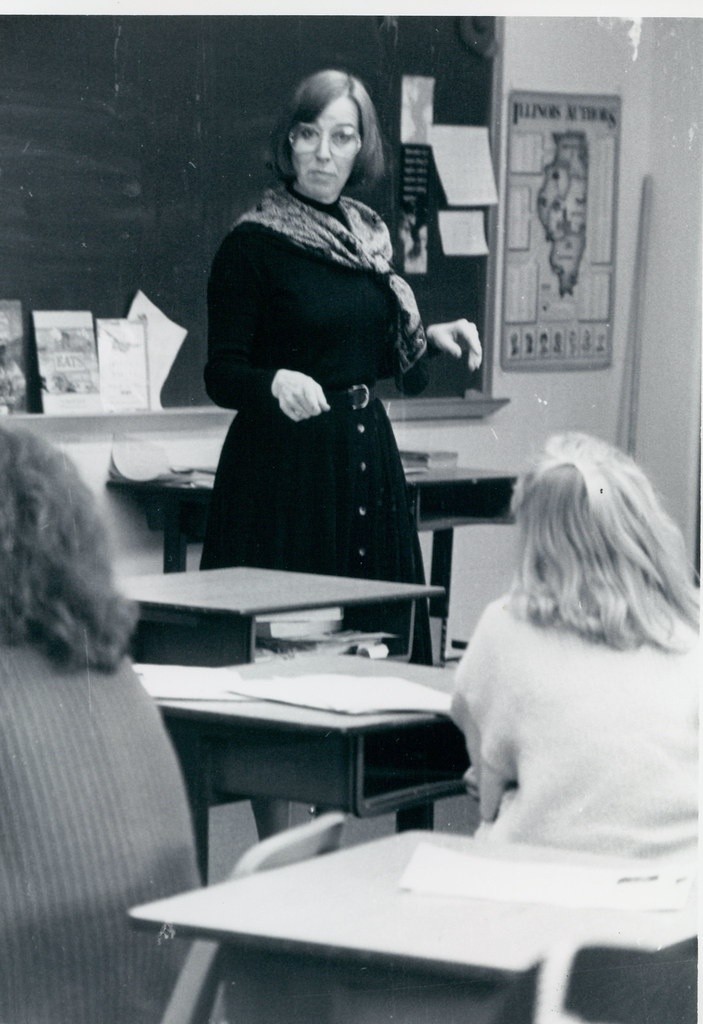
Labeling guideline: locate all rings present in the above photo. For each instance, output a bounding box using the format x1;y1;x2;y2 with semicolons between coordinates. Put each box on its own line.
295;410;303;416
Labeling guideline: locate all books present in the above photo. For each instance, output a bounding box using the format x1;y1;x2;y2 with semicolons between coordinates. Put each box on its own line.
254;607;384;660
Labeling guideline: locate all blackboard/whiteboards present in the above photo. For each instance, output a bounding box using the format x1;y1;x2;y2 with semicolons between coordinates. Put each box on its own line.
1;16;513;438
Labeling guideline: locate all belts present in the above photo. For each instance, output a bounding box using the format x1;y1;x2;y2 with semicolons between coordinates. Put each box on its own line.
326;384;379;408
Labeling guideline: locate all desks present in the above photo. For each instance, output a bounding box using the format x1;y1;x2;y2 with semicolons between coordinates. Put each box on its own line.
120;831;698;1024
160;655;471;889
105;463;524;668
120;564;447;675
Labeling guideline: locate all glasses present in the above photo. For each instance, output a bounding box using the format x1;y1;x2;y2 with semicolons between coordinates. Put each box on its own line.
288;122;363;158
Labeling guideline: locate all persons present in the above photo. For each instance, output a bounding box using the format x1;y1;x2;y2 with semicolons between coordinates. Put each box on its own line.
197;69;482;666
0;425;209;1024
450;432;702;872
